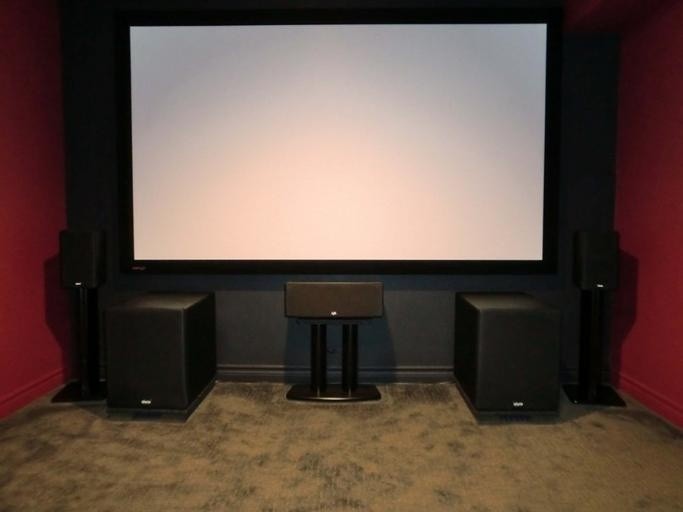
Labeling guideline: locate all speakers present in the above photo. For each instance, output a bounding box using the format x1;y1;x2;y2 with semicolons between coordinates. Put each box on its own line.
57;226;106;290
453;290;562;412
104;291;218;410
283;282;384;319
574;230;621;294
